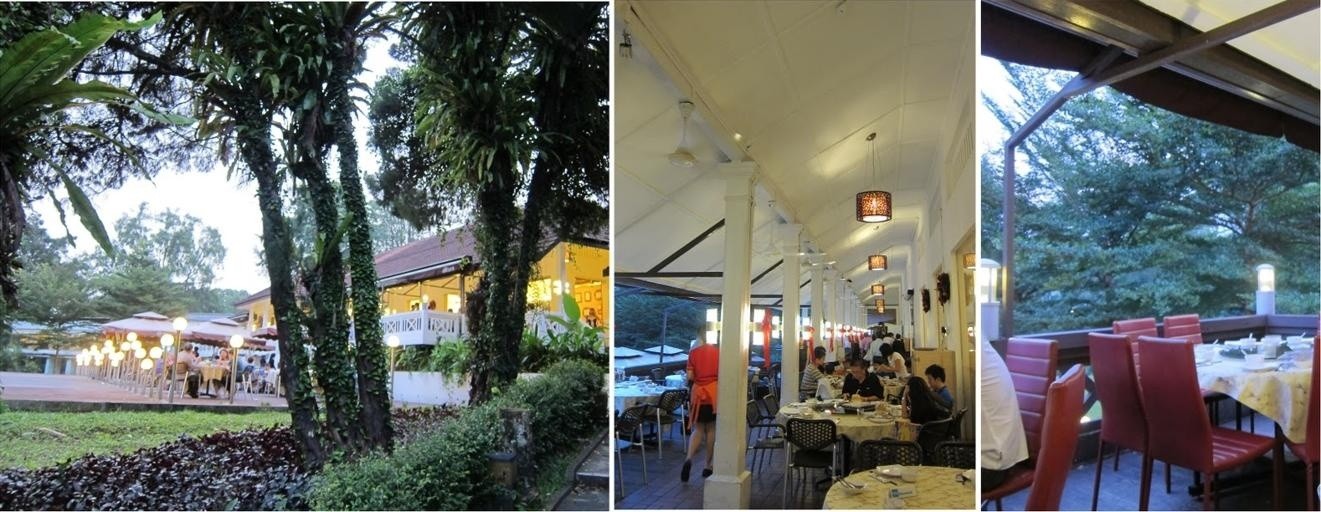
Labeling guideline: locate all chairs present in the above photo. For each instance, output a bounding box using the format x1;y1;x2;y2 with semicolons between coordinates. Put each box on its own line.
982;312;1320;510
158;346;320;400
615;340;977;509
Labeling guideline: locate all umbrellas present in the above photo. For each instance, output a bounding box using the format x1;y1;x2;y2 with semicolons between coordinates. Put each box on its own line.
181;316;265;353
100;310;180;339
248;326;286;341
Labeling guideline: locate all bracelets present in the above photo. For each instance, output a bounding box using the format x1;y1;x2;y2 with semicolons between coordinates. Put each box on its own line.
899;396;906;401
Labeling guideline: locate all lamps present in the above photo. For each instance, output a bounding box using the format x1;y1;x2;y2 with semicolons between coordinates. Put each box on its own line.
665;96;895;350
422;292;429;310
1255;263;1277;315
982;256;1004;341
961;238;976;270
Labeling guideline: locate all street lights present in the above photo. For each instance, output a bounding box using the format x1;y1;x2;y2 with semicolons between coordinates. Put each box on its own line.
74;316;190;402
229;333;244;403
387;333;401;403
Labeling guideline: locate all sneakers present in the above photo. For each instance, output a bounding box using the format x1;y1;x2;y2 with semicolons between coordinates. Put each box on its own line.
187;389;231;398
702;467;713;478
680;458;693;483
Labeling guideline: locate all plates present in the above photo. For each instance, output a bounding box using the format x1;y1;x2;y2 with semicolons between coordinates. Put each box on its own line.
841;462;917;495
963;469;976;482
615;382;672;395
785;398;903;423
1195;339;1316;371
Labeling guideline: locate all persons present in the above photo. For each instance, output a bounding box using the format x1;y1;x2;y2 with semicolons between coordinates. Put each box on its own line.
980;330;1031;493
428;301;437;330
798;317;910;408
677;322;719;483
447;308;453;313
155;344;278;399
526;302;535;330
415;302;419;312
585;307;600;331
898;376;951;427
410;304;414;312
925;361;956;408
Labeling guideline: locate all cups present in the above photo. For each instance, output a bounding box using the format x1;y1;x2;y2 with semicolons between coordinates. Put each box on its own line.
1240;334;1303;345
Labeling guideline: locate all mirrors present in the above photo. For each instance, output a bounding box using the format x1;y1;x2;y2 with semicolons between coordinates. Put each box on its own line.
953;231;979;447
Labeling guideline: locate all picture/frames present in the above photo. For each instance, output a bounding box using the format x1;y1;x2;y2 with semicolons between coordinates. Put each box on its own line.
574;289;602;320
